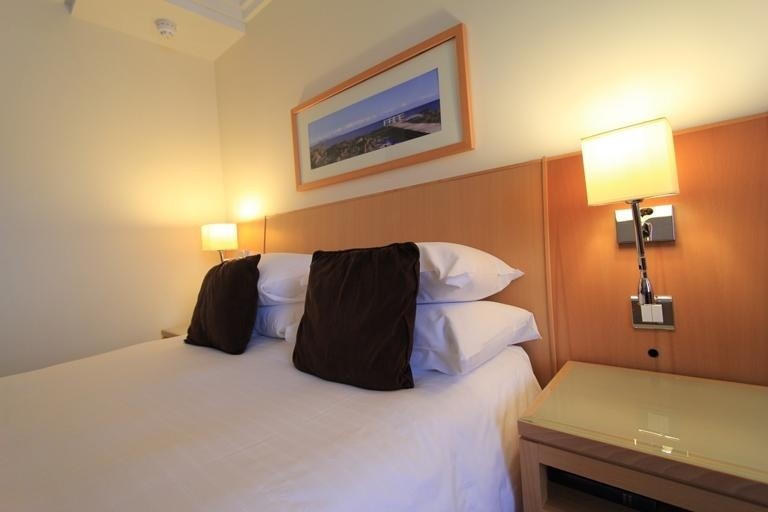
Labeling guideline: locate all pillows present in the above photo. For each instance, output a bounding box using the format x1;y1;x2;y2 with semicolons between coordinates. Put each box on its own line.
414;240;526;304
257;252;313;306
410;299;542;378
183;254;261;355
255;305;306;340
293;242;420;392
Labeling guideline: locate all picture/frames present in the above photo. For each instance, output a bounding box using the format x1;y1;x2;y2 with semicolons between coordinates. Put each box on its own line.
290;24;476;193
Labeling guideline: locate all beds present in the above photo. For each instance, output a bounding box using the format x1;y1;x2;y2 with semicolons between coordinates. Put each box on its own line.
0;327;542;512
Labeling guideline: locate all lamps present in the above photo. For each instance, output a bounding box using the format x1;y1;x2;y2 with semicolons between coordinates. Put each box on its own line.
200;223;238;263
581;118;679;304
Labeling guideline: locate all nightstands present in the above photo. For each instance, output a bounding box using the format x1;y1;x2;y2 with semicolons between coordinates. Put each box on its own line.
161;327;180;339
516;361;767;512
